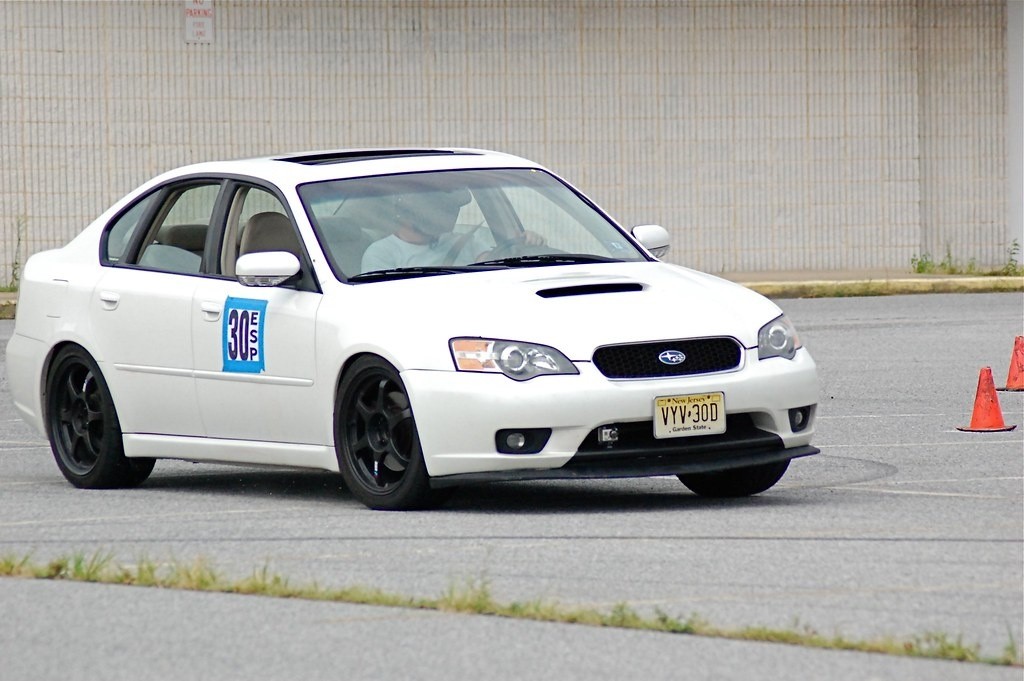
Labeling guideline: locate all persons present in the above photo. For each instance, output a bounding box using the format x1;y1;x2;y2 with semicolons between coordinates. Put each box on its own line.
360;188;547;274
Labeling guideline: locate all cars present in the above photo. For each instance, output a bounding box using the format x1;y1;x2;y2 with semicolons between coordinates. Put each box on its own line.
5;147;823;519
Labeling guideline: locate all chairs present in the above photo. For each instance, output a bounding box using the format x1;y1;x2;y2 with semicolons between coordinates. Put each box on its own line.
154;223;222;274
317;215;374;277
239;211;318;292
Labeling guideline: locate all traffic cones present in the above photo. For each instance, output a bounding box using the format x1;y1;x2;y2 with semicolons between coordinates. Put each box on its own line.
957;364;1019;433
996;335;1024;390
